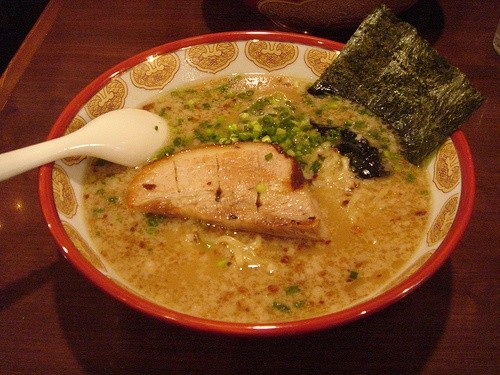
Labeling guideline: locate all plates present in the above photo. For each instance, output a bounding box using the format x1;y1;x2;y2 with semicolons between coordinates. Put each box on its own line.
38;30;473;338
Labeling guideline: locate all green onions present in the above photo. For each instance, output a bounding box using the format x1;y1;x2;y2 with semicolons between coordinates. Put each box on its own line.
80;74;429;311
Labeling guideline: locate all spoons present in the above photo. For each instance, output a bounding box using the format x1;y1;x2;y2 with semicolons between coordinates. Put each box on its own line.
1;108;169;182
